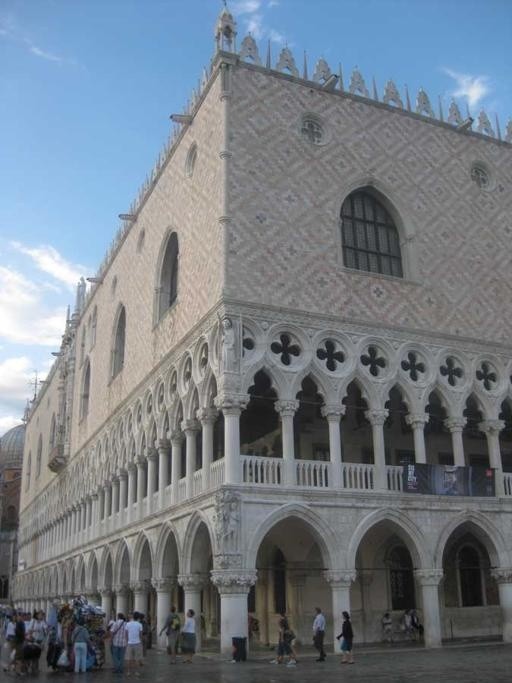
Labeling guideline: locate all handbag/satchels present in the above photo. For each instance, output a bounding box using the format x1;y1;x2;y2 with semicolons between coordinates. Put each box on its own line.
169;616;180;630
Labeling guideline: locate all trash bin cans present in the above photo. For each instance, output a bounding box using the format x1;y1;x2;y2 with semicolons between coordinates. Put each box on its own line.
232;637;247;660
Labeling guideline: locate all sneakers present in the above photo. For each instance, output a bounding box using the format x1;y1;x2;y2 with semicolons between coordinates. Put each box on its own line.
339;659;356;665
315;654;328;662
269;657;299;665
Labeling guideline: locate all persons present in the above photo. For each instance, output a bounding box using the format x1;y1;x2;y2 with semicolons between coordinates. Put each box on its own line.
159;606;181;663
312;607;326;662
278;615;296;662
219;318;240;374
0;604;149;677
269;611;296;664
411;610;424;634
403;608;413;632
382;613;392;629
181;608;196;663
336;611;354;663
